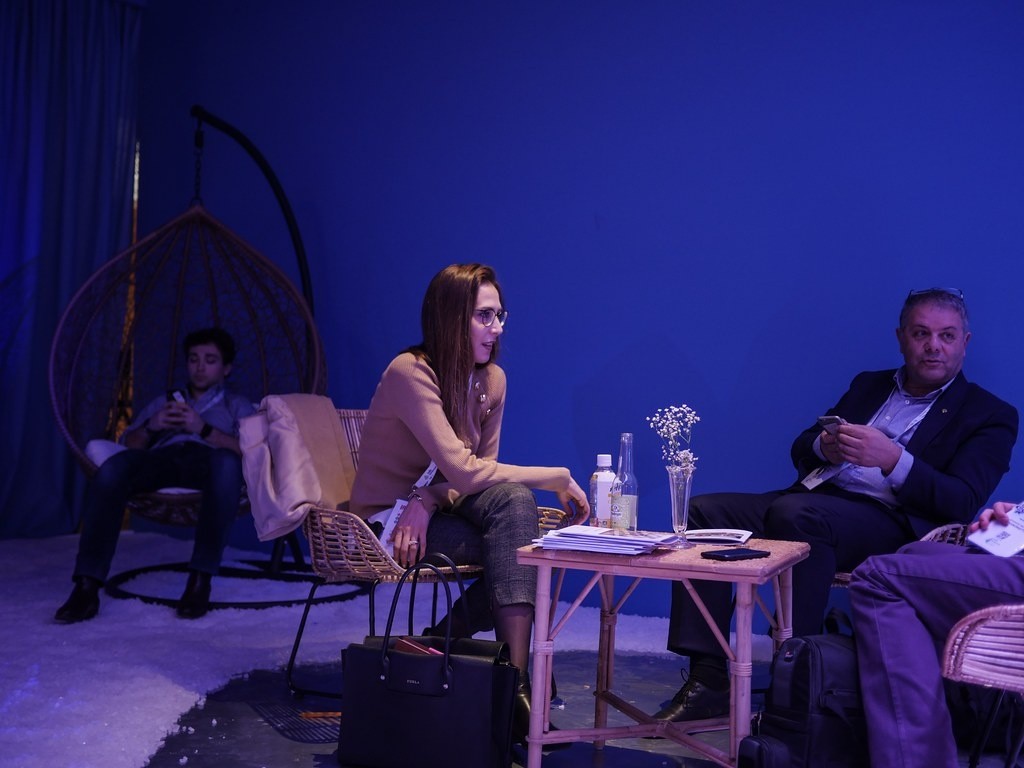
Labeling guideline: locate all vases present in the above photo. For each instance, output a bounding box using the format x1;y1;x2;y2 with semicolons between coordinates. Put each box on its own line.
660;465;696;548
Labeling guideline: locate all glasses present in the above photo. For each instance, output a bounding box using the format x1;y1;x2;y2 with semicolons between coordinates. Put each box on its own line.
472;307;508;328
900;286;967;328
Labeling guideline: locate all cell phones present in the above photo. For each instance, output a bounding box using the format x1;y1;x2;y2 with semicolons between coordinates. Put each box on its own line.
700;548;771;562
166;389;188;411
818;416;845;435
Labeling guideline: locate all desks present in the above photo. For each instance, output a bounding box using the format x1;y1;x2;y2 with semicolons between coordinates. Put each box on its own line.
517;532;810;768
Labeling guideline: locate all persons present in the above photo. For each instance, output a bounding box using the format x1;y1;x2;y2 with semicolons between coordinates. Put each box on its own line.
642;285;1018;736
346;263;589;753
847;501;1024;768
54;328;258;623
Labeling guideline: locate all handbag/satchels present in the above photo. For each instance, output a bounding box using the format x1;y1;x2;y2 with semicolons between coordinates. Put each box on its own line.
336;552;515;768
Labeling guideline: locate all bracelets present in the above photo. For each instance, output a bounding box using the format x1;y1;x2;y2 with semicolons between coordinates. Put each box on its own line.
200;422;213;439
414;495;422;500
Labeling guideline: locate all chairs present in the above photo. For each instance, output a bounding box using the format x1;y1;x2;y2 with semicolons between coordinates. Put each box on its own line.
287;409;572;698
941;605;1024;768
51;104;377;609
734;523;974;633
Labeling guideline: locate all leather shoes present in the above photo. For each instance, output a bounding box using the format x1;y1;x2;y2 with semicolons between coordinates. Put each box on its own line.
53;582;100;620
177;574;213;618
515;678;573;749
638;668;730;739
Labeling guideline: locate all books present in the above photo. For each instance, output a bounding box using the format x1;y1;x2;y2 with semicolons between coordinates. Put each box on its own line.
684;529;753;546
531;523;681;555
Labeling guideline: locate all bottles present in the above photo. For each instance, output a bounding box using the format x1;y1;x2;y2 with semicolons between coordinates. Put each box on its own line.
612;433;638;536
589;454;616;528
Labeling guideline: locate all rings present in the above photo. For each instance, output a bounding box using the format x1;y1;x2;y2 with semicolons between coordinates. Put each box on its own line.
409;541;418;545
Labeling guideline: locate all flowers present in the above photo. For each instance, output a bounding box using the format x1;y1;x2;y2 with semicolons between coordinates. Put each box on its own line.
646;403;701;526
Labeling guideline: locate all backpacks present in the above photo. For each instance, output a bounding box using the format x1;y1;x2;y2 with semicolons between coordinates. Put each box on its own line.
736;637;871;768
